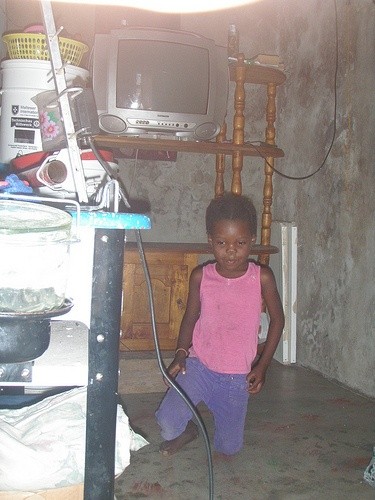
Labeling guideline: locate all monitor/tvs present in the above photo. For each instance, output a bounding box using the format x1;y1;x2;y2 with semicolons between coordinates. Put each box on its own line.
92;26;229;142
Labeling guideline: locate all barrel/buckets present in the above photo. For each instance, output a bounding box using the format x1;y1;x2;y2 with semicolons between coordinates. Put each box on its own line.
1;59;89;164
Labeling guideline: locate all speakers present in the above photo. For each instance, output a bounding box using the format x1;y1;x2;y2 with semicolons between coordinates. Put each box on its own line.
73;87;100;136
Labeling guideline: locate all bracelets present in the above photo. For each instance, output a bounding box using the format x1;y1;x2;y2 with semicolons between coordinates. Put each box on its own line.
175;348;188;357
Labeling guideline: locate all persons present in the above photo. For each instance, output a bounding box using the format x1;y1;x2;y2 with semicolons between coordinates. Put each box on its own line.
155;192;285;456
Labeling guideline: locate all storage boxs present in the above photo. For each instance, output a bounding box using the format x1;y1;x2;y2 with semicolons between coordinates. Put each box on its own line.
3;33;89;66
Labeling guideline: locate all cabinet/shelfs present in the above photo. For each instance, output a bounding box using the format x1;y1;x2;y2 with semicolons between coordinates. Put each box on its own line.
0;208;153;500
93;23;285;351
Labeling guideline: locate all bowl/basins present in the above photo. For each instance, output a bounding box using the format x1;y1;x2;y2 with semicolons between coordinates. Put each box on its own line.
11;151;53;172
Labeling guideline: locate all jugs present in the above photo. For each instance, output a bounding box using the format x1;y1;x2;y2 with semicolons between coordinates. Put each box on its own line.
31;89;81;151
